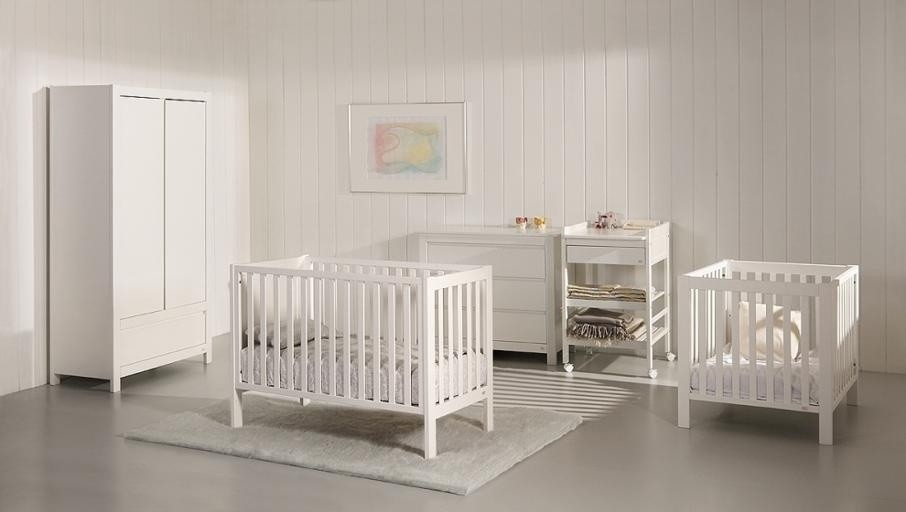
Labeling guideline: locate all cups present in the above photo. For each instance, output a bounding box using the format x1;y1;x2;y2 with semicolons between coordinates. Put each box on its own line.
534;216;546;235
516;216;528;234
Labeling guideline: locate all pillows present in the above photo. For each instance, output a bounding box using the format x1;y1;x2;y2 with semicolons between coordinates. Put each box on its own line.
246;317;345;348
738;300;803;359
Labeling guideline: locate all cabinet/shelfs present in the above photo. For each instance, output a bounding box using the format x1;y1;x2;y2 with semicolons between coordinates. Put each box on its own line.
561;217;675;378
47;85;211;393
416;224;561;366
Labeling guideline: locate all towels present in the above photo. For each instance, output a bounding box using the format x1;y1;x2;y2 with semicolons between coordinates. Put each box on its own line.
566;284;619;297
615;281;656;301
569;317;643;339
570;306;630;339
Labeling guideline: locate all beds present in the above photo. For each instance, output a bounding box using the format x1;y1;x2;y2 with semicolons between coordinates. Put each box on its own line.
677;258;860;444
227;253;493;459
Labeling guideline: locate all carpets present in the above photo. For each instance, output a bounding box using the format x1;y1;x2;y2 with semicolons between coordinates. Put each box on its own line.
122;394;583;494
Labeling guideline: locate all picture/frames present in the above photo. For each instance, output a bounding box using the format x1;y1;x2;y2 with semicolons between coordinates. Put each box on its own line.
346;101;467;194
